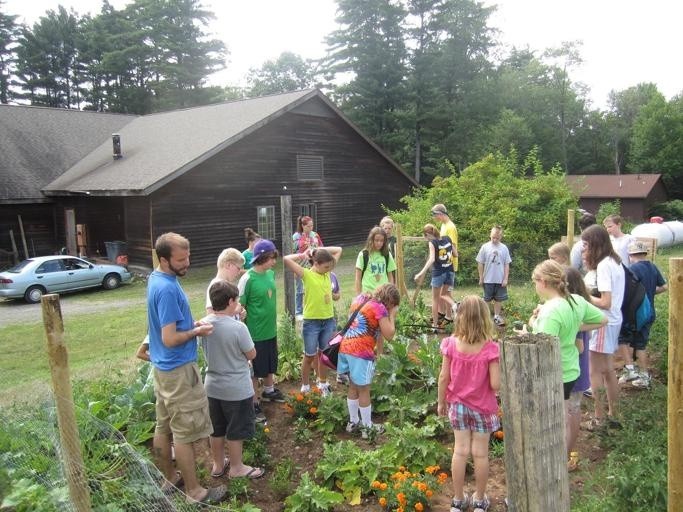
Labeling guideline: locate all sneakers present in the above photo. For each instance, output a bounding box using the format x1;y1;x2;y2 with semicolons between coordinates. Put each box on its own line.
253;401;268;423
260;387;290;404
300;384;311;393
345;420;362;433
449;492;469;512
317;383;332;397
618;364;639;383
580;419;606;433
607;414;622;429
494;314;506;326
470;492;490;512
632;371;651;388
361;424;385;439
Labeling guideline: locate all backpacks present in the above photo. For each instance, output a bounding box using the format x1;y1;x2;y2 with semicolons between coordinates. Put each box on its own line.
320;296;374;370
330;270;341;301
620;262;654;361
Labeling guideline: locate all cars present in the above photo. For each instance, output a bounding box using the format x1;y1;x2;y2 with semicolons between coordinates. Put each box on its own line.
0;252;133;305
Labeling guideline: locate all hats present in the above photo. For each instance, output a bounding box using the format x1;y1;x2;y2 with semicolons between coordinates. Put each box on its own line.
249;239;277;265
625;239;649;256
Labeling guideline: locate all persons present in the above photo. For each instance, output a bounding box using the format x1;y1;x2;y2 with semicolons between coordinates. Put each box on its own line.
414;224;461;332
205;248;254;377
438;294;504;512
136;334;150;362
330;272;341;323
548;243;570;267
284;247;342;398
561;266;593;433
356;226;397;360
617;240;666;390
431;204;459;327
380;216;398;260
603;214;637;268
146;232;228;507
198;281;265;480
580;224;626;431
512;260;608;472
240;227;261;275
568;214;597;277
236;240;289;423
319;282;401;439
291;216;324;321
475;226;512;326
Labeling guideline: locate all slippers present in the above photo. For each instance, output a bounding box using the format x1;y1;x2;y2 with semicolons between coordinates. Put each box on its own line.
430;311;446;320
159;470;184;495
437;317;454;326
185;484;229;508
210;457;230;477
228;466;265;479
